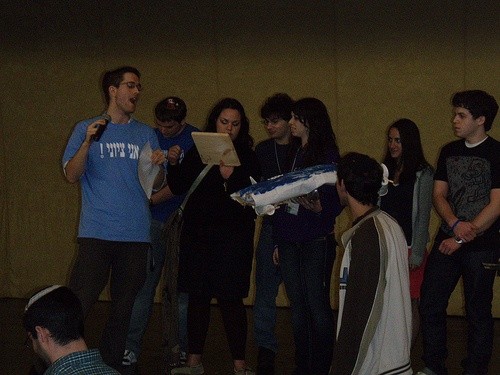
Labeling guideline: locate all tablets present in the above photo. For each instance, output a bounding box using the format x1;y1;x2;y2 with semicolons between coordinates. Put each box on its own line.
191;132;241;166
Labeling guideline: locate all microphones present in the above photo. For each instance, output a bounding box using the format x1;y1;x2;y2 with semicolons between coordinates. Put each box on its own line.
93;114;111;142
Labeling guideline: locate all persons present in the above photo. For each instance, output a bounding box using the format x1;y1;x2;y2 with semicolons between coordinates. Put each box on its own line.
28;65;169;375
416;89;500;375
121;93;434;375
25;284;120;375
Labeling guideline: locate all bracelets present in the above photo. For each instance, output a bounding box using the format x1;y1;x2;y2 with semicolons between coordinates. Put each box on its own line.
454;235;466;247
148;198;154;208
450;220;462;233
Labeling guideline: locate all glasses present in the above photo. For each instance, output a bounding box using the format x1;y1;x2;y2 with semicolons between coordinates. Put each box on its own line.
120;81;144;91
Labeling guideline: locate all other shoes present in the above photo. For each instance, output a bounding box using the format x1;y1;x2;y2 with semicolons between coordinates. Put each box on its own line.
170;364;205;375
122;349;138;365
233;367;256;375
